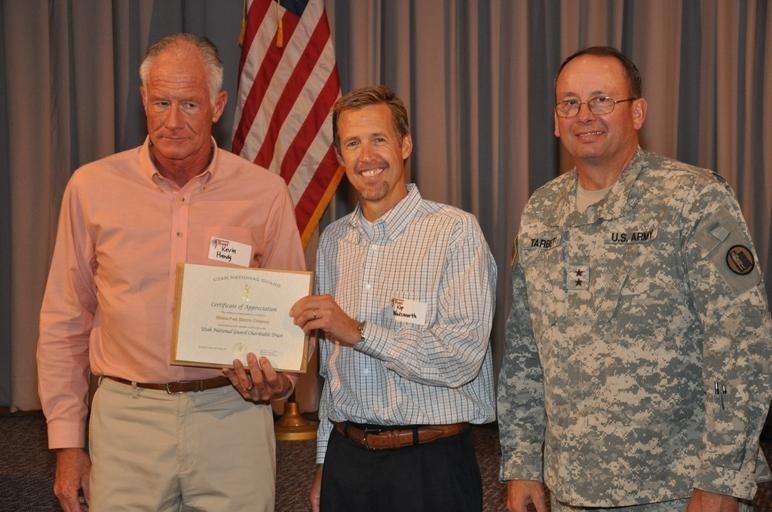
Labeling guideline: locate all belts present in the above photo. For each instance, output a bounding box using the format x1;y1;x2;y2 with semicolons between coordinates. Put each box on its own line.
335;421;466;450
104;373;231;394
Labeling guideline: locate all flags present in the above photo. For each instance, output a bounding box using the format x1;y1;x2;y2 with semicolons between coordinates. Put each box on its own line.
229;0;346;253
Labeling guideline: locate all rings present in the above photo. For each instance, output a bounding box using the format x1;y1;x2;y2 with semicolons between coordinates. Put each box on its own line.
242;375;253;393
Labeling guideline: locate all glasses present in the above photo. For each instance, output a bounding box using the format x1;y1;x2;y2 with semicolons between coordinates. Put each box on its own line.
555;96;633;118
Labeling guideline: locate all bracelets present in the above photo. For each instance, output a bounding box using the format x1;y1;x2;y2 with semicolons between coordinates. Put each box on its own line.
358;321;365;340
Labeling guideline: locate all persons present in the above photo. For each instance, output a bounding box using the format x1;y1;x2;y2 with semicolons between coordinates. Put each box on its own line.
32;27;316;511
288;80;500;512
494;44;772;512
